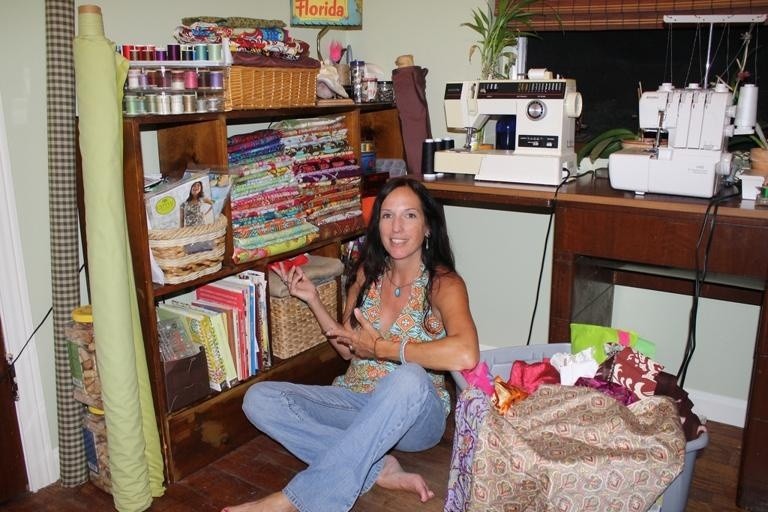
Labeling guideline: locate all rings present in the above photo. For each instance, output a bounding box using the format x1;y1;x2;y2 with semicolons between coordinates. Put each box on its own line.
349;344;353;351
281;279;287;284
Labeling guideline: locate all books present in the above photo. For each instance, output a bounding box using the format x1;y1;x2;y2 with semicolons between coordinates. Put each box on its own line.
155;270;272;392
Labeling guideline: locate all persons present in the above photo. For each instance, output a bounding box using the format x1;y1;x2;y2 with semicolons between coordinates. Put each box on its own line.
179;181;213;228
218;176;481;512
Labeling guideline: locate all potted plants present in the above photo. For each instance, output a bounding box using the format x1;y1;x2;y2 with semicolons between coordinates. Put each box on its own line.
459;1;568;151
571;126;671;171
720;72;768;194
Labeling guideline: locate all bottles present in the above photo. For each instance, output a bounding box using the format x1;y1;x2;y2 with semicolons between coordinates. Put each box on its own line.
378;81;394;102
350;60;365;103
362;76;378;102
70;304;112;493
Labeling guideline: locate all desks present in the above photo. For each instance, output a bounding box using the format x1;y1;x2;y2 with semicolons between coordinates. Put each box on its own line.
421;171;767;511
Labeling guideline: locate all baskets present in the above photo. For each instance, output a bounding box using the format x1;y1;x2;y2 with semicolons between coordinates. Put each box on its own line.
145;211;229;287
222;65;322;113
267;277;340;361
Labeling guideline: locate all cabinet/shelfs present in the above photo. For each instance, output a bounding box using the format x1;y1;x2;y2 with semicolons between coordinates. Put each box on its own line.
73;101;408;486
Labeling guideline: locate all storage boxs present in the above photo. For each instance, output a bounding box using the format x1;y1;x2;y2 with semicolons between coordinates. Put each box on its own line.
448;341;710;510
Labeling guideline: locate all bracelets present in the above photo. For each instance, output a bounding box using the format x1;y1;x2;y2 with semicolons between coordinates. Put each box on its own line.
400;338;410;364
373;336;384;365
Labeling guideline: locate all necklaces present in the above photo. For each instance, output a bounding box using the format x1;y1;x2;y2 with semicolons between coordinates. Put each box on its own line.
386;268;423;297
